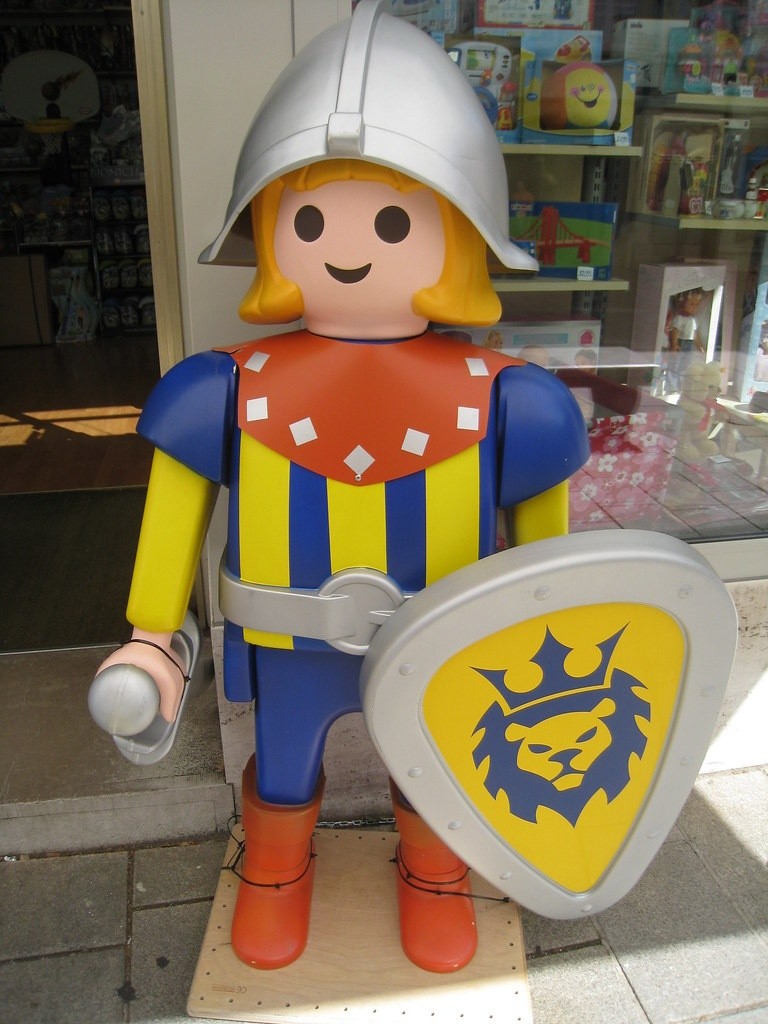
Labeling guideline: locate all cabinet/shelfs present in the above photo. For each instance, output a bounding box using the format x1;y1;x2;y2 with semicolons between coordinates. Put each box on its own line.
0;5;156;335
487;95;768;291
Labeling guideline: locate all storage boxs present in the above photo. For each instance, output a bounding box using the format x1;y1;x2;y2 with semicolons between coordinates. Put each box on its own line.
541;357;685;525
0;255;54;348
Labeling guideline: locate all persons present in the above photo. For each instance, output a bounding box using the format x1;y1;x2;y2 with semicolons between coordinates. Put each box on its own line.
90;0;593;973
666;287;705;394
574;349;597;374
487;331;503;349
